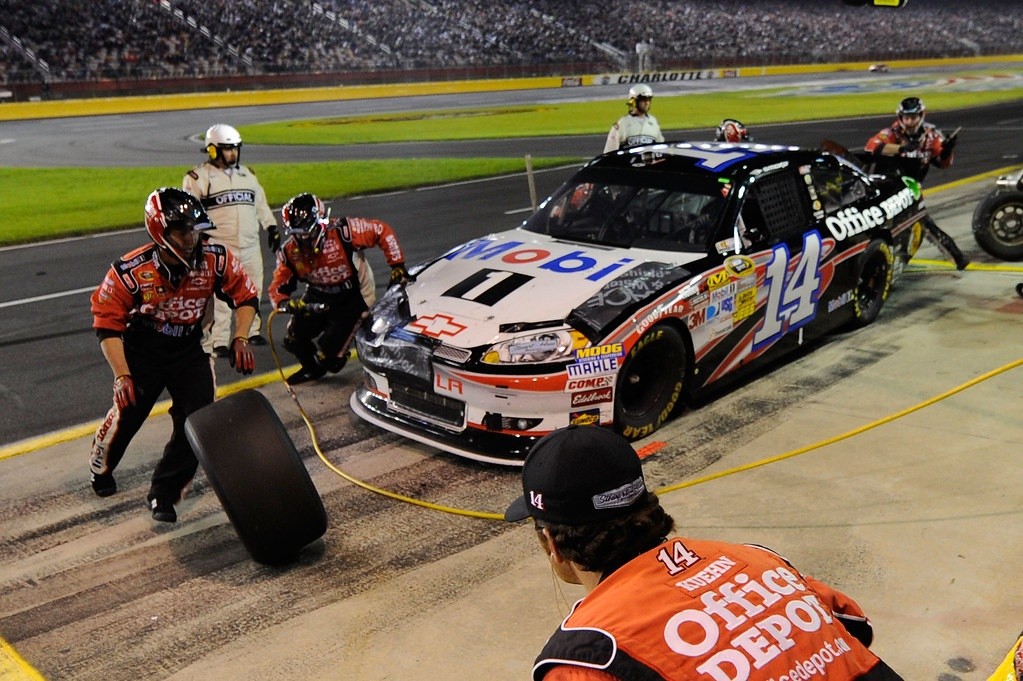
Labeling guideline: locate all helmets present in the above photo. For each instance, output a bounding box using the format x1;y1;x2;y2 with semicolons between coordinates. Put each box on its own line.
628;83;654;100
896;97;926;135
283;193;329;251
145;187;217;269
204;124;244;161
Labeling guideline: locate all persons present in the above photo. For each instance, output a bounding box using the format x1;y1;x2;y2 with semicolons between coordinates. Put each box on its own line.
861;97;970;271
603;83;666;155
503;424;905;681
713;119;754;143
268;192;407;385
182;123;281;357
0;0;1023;96
88;186;259;523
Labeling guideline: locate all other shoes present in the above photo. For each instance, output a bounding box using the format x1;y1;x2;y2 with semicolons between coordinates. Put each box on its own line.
213;345;229;359
248;335;267;346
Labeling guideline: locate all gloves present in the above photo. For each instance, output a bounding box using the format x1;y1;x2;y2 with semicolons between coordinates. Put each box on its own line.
228;338;256;375
941;134;959;153
387;263;415;292
278;297;308;314
897;143;921;158
113;374;137;413
265;225;282;253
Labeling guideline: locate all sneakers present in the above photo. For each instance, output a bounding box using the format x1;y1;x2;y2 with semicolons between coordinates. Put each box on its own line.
308;349;330;380
287;367;311;386
147;492;178;522
90;468;117;498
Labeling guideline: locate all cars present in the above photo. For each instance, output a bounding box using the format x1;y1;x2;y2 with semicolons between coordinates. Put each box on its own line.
972;168;1023;262
349;142;927;467
869;64;888;73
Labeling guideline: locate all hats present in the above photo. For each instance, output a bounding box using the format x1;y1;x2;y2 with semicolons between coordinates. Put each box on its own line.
504;423;649;524
